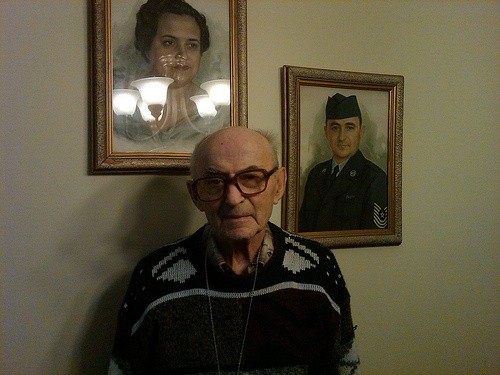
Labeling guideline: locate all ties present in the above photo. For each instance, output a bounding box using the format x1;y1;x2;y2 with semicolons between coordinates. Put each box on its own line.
328;165;339;189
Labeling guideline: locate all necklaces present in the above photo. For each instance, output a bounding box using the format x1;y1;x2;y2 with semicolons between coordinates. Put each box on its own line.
204;222;261;375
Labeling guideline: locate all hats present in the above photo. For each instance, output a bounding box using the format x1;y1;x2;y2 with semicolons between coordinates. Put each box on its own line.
326;93;361;120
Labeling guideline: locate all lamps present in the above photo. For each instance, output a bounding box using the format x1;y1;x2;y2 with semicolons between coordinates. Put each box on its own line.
111;55;230;143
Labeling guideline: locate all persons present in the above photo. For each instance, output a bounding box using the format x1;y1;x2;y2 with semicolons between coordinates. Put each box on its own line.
107;125;362;375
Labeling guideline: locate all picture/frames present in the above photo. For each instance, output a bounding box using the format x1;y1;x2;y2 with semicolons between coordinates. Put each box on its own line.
87;0;249;175
281;64;404;248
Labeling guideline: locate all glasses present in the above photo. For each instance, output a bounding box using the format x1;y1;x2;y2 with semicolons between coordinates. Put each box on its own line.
192;166;279;203
